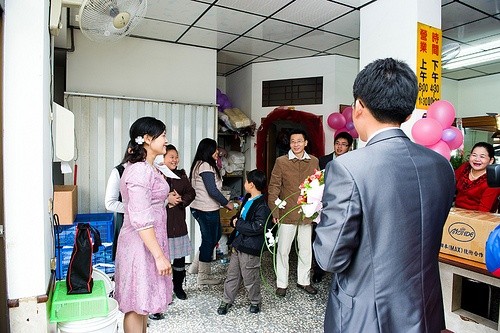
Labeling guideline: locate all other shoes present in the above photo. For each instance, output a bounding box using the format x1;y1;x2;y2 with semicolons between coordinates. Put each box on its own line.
250;302;261;313
217;301;232;315
276;286;288;297
297;282;318;294
151;314;161;321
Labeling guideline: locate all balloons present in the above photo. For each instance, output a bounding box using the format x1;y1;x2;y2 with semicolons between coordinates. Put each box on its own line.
440;126;464;150
327;107;359;138
412;118;442;146
427;101;455;127
217;89;233;112
430;140;451;161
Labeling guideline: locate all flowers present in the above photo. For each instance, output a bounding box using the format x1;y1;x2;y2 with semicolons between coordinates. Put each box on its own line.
258;168;324;295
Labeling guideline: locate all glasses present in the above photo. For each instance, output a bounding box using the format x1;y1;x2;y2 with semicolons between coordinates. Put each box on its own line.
351;99;364;110
335;142;349;147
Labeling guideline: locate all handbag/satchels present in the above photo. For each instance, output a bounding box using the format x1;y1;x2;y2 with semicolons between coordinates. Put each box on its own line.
66;222;103;294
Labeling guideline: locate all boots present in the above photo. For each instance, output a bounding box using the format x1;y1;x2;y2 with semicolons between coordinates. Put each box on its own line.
187;250;200;273
197;261;223;285
172;268;187;300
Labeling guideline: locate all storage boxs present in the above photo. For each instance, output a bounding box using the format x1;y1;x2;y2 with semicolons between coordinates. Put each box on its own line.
52;281;108;323
439;207;500;262
55;213;115;248
52;185;78;226
55;244;116;280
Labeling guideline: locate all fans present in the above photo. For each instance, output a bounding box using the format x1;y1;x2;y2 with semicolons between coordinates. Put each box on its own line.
49;0;148;43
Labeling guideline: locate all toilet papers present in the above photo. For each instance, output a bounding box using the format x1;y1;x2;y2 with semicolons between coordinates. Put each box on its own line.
225;151;245;174
226;107;250;128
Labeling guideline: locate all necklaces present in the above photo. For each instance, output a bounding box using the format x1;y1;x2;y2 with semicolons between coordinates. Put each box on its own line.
471;168;486;179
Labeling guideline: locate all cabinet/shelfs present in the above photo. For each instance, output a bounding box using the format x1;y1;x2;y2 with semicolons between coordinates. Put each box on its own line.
216;130;244;256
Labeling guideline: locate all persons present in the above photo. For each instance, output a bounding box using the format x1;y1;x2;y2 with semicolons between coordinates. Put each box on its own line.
313;57;455;333
188;138;233;285
312;132;353;283
454;142;500;213
160;144;196;300
113;117;171;333
104;140;142;262
217;169;273;315
267;129;321;297
